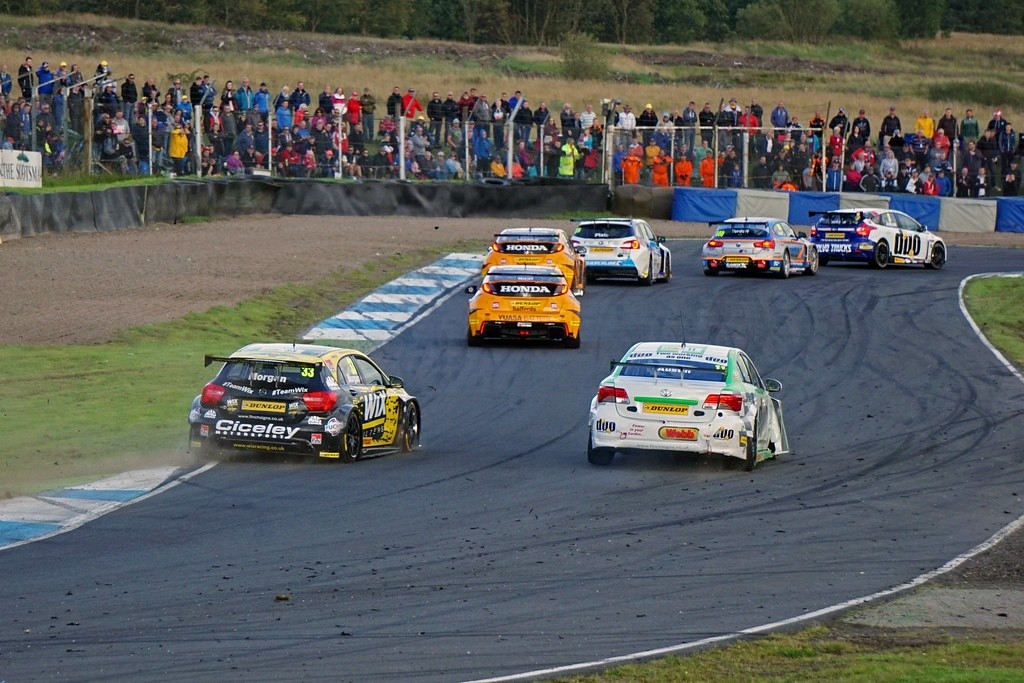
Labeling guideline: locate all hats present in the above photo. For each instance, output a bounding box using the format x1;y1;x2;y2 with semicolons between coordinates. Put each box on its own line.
417;116;424;120
141;97;147;101
784;145;789;149
352;91;358;96
663;112;669;117
646;103;652;108
839;107;844;113
60;62;66;66
650;138;655;142
729;97;738;103
101;61;108;66
181;95;188;100
409;88;414;92
833;159;840;163
453;119;459;124
203;147;211;152
248;145;254;148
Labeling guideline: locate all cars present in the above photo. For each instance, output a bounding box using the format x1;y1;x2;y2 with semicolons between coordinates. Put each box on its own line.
568;218;672;285
701;217;820;276
463;264;584;348
588;341;789;471
809;208;949;269
481;228;585;293
189;343;423;462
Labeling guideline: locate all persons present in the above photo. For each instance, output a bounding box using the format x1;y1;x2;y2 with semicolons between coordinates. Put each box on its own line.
696;97;763;188
0;57;88;177
407;88;523;179
271;81;349;177
606;101;698;186
845;105;964;197
753;102;850;191
190;75;286;177
343;87;424;179
956;109;1021;197
512;100;602;180
92;60;213;179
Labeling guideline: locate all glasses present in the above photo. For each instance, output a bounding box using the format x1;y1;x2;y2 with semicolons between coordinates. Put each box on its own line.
451;155;456;157
258;125;263;126
130;77;134;79
482;96;486;98
